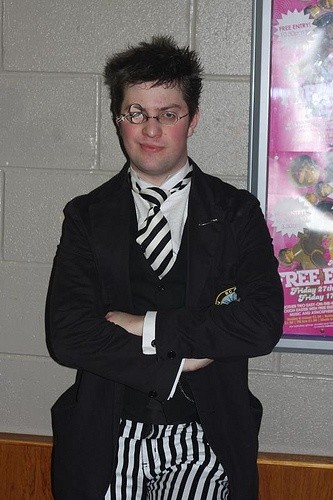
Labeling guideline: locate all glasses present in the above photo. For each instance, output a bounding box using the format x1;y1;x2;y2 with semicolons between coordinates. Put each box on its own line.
115;103;189;125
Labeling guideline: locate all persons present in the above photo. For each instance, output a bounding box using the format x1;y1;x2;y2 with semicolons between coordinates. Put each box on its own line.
44;33;284;500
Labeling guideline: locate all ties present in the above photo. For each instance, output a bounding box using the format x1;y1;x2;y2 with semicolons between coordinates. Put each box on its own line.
130;171;194;279
